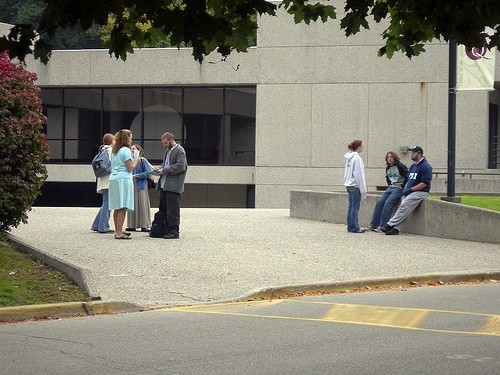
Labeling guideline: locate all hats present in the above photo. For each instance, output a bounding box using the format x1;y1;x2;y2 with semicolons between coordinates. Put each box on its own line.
408;146;423;155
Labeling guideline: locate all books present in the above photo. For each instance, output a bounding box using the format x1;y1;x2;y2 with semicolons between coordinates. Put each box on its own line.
146;161;161;175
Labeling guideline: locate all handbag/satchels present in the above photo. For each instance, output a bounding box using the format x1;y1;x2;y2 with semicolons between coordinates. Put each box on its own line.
147;177;156;189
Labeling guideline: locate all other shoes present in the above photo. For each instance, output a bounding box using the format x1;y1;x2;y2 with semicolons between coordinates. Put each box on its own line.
364;225;374;231
381;224;391;232
385;228;399;235
114;231;132;239
164;235;179;239
374;226;382;233
347;227;365;233
141;227;151;232
126;227;136;231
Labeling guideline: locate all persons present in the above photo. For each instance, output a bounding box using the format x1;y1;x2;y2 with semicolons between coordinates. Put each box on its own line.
109;129;141;239
364;151;409;232
125;144;152;232
381;146;432;235
344;140;366;233
90;134;115;233
149;132;188;239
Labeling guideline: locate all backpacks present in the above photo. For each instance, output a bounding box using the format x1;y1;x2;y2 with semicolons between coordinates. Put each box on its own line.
92;147;112;177
